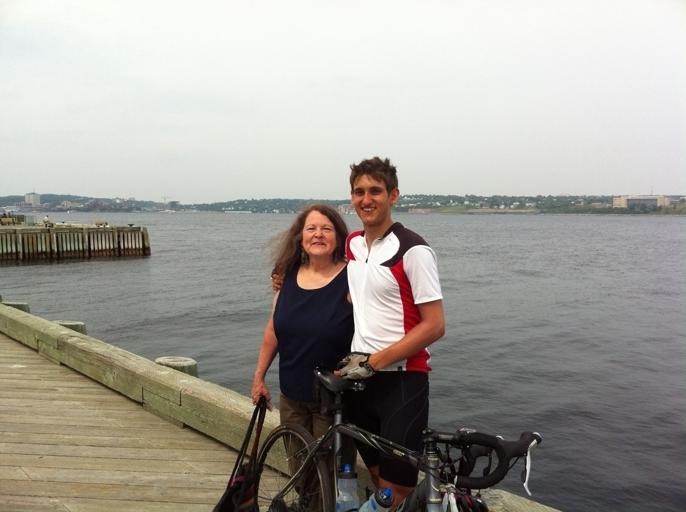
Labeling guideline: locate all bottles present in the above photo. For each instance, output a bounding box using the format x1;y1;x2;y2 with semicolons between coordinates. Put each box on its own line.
337;465;360;508
361;489;395;507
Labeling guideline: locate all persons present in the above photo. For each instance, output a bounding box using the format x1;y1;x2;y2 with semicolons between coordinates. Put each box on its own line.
252;205;355;512
273;156;446;512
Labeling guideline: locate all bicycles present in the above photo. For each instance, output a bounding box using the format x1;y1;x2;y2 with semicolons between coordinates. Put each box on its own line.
258;366;543;511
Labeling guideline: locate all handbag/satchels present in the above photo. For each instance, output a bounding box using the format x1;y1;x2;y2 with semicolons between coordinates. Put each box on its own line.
212;462;259;512
